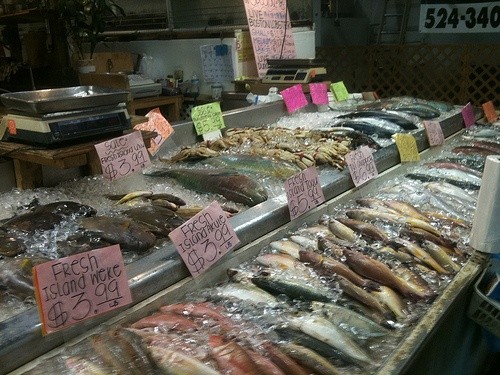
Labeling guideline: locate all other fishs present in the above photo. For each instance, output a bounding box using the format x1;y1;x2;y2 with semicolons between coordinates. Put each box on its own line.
0;102;499;375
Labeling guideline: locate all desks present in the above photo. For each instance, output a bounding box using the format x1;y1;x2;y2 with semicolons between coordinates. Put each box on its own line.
78;73;183;124
0;139;158;189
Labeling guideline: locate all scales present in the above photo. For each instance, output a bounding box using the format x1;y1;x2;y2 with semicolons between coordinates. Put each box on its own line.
0;86;132;147
262;58;327;84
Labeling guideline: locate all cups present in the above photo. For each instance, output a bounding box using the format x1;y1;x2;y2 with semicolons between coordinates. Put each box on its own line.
78;60;88;74
87;59;97;73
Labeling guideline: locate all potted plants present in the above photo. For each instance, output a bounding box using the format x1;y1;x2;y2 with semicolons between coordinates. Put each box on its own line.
57;0;126;72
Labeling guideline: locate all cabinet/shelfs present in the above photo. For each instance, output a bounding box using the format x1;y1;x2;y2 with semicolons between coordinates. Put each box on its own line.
0;0;71;93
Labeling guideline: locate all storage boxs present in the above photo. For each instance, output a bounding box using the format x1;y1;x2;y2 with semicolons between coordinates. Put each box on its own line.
468;258;500;339
